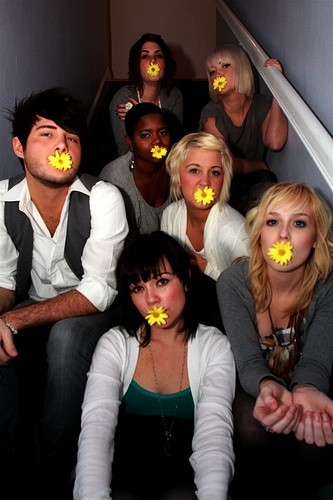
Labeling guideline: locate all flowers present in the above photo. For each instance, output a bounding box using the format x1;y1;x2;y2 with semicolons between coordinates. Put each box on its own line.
145;304;168;326
150;146;168;158
48;150;73;170
194;185;215;206
213;77;227;90
267;242;294;266
147;63;160;76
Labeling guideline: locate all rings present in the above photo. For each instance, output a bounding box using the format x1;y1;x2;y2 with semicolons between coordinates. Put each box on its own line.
125;101;133;110
266;427;273;432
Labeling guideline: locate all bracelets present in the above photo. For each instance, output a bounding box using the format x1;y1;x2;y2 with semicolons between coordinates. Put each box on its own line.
1;317;17;334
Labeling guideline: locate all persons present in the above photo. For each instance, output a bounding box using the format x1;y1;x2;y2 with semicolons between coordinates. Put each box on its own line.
73;230;236;500
0;90;129;429
99;34;288;279
216;181;333;448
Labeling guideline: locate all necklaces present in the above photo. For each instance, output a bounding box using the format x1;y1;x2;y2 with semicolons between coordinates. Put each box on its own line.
152;87;159;103
129;157;134;171
268;306;295;376
149;344;186;441
